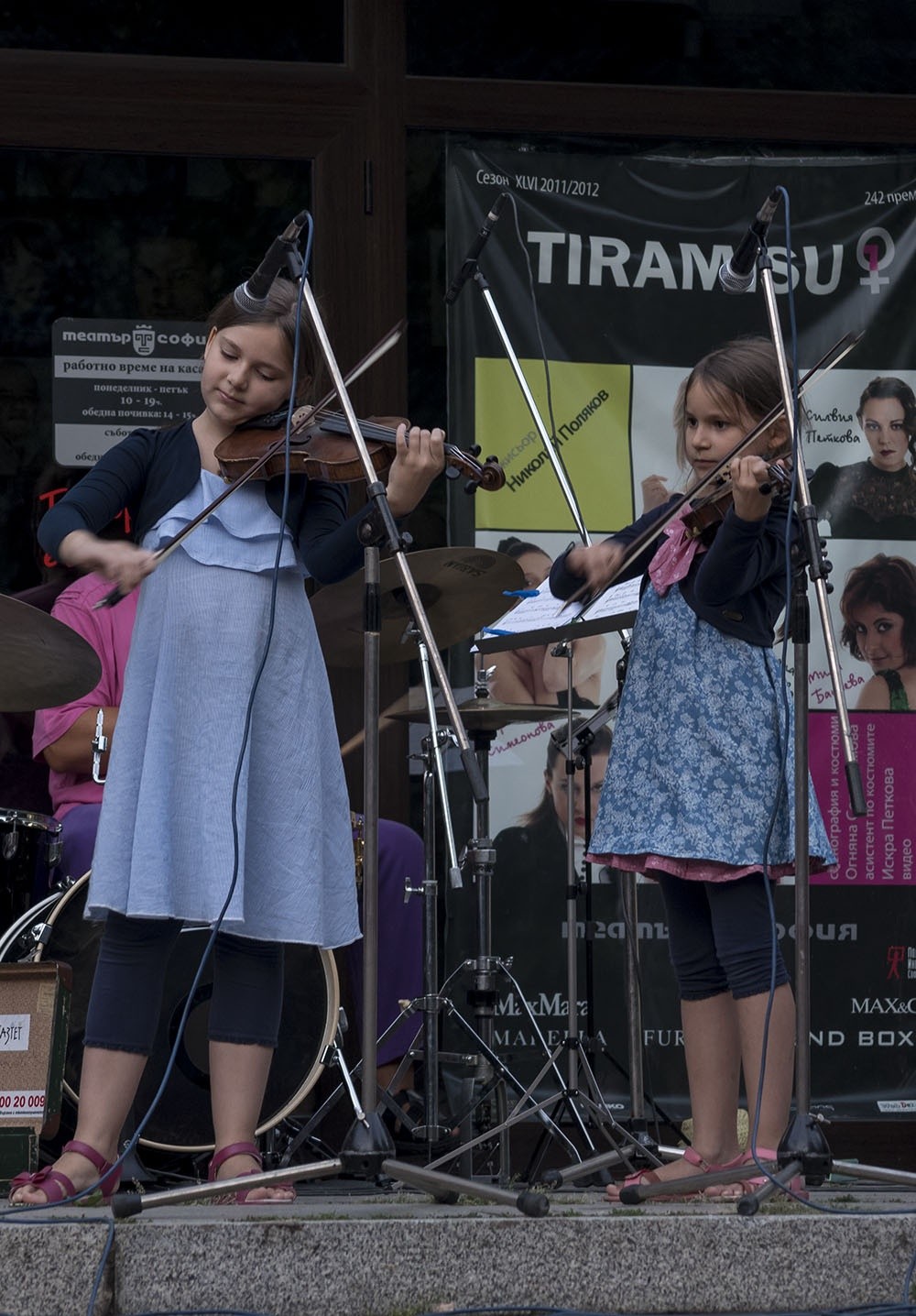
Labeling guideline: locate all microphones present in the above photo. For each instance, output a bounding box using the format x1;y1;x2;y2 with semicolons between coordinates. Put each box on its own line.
720;187;784;295
444;192;509;306
235;211;308;315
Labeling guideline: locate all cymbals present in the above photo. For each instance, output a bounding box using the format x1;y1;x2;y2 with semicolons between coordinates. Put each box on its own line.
465;606;641;656
313;547;527;666
0;594;102;712
378;696;575;730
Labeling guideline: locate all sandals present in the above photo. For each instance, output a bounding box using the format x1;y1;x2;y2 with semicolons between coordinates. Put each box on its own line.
381;1088;461;1150
207;1142;297;1203
603;1147;809;1203
9;1141;122;1205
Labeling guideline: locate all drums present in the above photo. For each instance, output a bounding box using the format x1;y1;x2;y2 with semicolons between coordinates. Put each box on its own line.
1;806;68;939
1;865;343;1154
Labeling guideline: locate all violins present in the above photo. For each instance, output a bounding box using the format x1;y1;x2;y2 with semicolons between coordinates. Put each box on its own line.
676;459;798;533
212;398;508;496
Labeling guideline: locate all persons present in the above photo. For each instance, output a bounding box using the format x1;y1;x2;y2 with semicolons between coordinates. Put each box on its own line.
549;337;838;1202
11;278;445;1205
839;552;916;711
807;376;916;540
484;476;671;884
31;567;463;1155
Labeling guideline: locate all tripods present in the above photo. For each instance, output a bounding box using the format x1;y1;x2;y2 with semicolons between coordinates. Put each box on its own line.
111;235;916;1219
